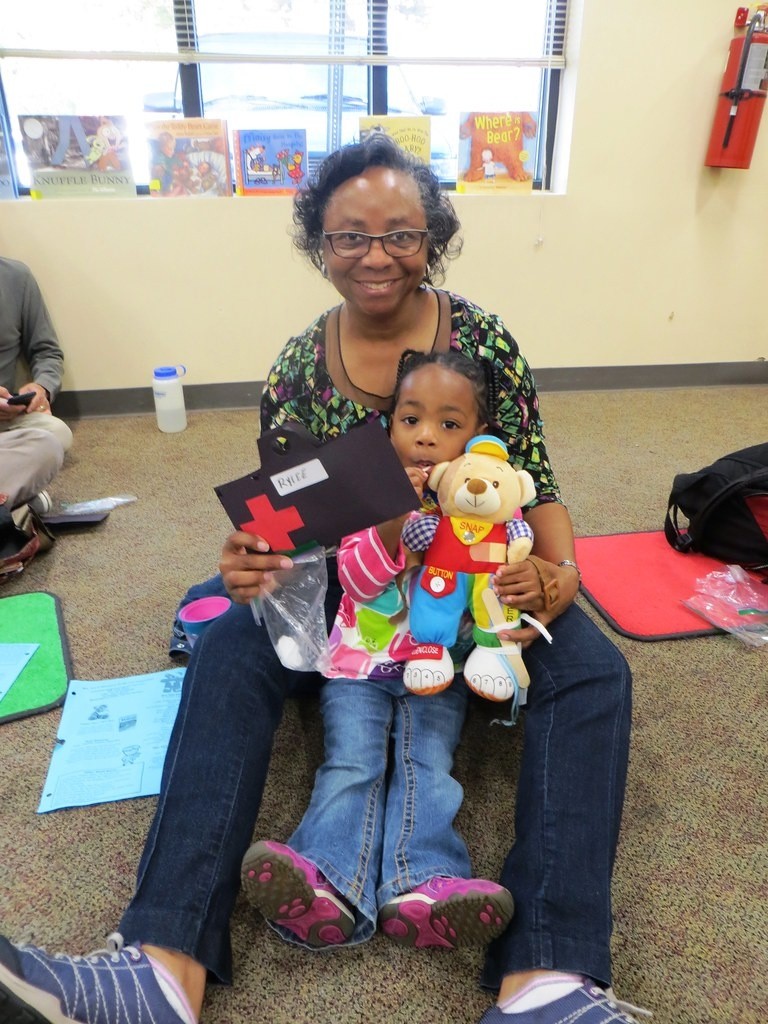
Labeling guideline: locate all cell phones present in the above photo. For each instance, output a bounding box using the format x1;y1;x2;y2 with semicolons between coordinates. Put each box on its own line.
6;391;36;411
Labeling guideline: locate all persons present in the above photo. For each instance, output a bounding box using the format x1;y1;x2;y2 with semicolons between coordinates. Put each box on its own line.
0;136;646;1024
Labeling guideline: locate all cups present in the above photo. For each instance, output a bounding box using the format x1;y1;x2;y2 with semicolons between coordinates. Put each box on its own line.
151;363;189;434
178;593;232;650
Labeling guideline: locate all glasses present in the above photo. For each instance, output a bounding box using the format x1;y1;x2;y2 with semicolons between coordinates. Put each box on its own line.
322;227;430;258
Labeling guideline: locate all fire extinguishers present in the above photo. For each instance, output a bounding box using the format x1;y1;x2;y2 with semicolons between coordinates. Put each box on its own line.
706;6;768;169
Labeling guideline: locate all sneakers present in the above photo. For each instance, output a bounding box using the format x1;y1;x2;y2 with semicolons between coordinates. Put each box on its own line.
243;841;356;947
379;874;513;950
27;489;52;515
0;929;188;1024
477;977;654;1024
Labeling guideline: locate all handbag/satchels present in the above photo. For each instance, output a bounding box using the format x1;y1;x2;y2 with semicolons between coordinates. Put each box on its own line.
665;441;768;586
